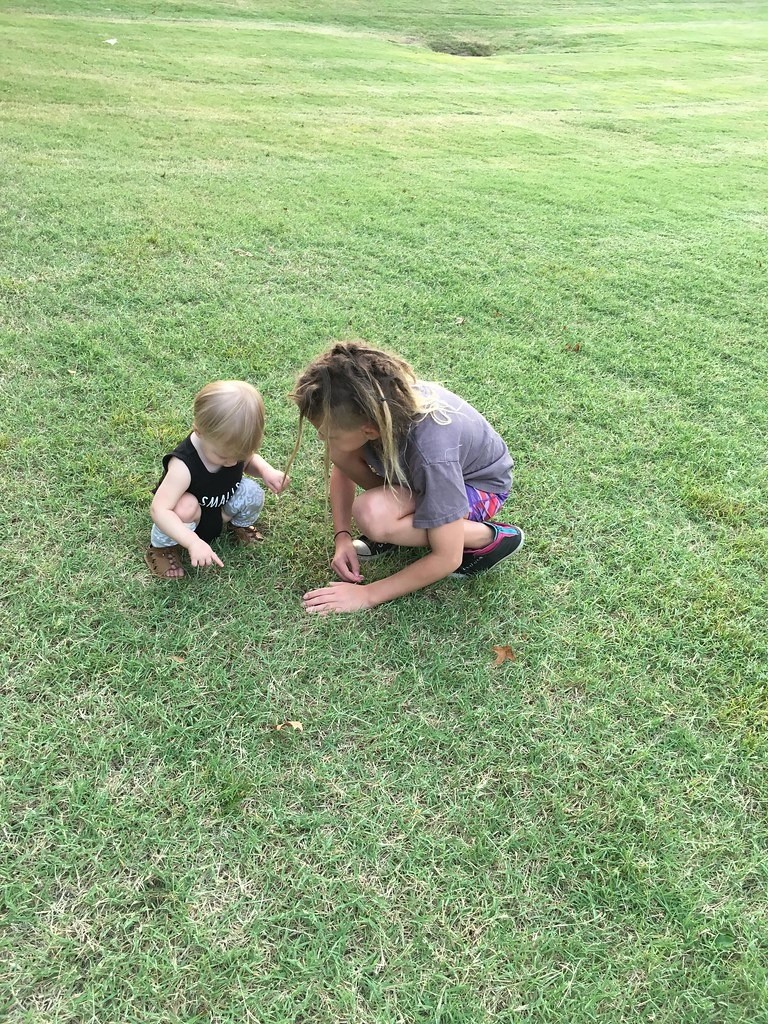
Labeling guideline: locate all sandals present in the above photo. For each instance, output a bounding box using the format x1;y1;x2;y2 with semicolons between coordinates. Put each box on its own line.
228;522;263;544
144;543;185;579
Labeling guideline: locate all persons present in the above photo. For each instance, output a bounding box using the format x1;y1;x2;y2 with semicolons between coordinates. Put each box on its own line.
146;380;292;579
295;341;526;617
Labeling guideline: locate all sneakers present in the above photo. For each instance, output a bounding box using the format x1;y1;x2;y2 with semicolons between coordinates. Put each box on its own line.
449;522;524;579
350;534;396;560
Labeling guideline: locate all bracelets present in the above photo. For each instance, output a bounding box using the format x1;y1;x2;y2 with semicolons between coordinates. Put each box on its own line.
333;530;353;542
